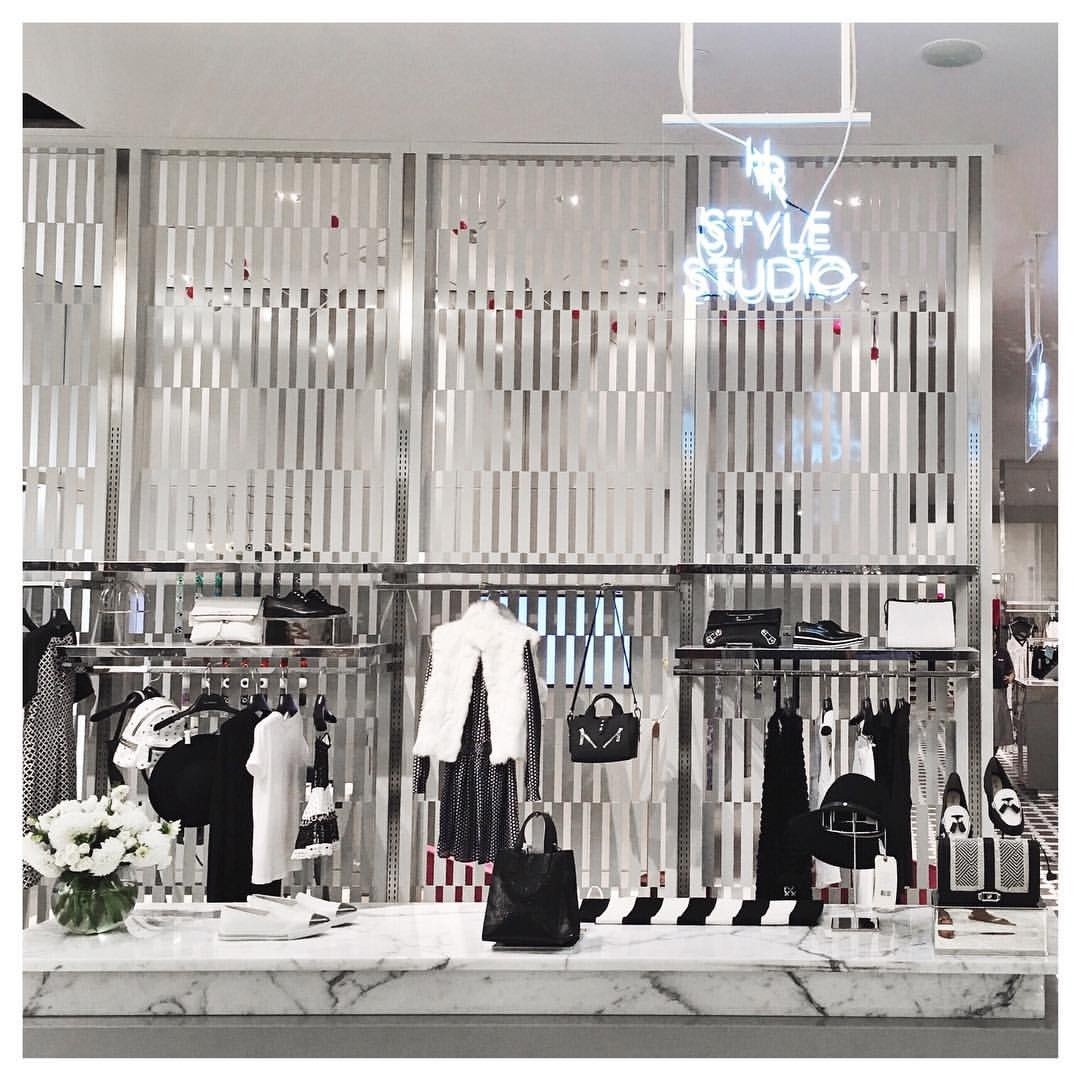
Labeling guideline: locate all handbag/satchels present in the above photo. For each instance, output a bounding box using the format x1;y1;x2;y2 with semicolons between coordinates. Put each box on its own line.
702;608;782;647
568;693;642;763
937;839;1041;907
188;596;265;645
482;812;582;948
993;648;1015;689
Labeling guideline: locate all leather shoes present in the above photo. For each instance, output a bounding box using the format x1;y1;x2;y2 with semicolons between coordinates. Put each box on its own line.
940;773;973;840
983;757;1025;835
264;590;326;617
793;620;863;648
305;590;347;615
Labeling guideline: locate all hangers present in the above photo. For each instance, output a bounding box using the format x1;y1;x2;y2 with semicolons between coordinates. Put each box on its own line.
313;666;338;732
821;668;829;712
89;663;165;723
879;671;889;711
23;608;38;632
152;663;242;732
49;582;70;625
782;669;793;710
863;671;869;711
220;666;274;734
274;665;299;719
479;581;506;610
894;670;906;709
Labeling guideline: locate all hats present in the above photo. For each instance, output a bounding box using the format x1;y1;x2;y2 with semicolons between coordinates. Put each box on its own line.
794;774;903;869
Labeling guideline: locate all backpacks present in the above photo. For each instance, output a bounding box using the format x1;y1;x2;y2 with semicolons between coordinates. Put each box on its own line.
111;689;186;767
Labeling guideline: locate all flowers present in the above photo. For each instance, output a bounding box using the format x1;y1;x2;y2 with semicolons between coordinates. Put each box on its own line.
23;784;181;930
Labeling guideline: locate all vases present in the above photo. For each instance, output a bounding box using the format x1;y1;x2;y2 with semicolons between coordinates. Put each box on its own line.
48;861;140;935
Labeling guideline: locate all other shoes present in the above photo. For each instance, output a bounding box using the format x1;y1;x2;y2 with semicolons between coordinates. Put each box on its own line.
245;892;357;918
219;903;332;940
